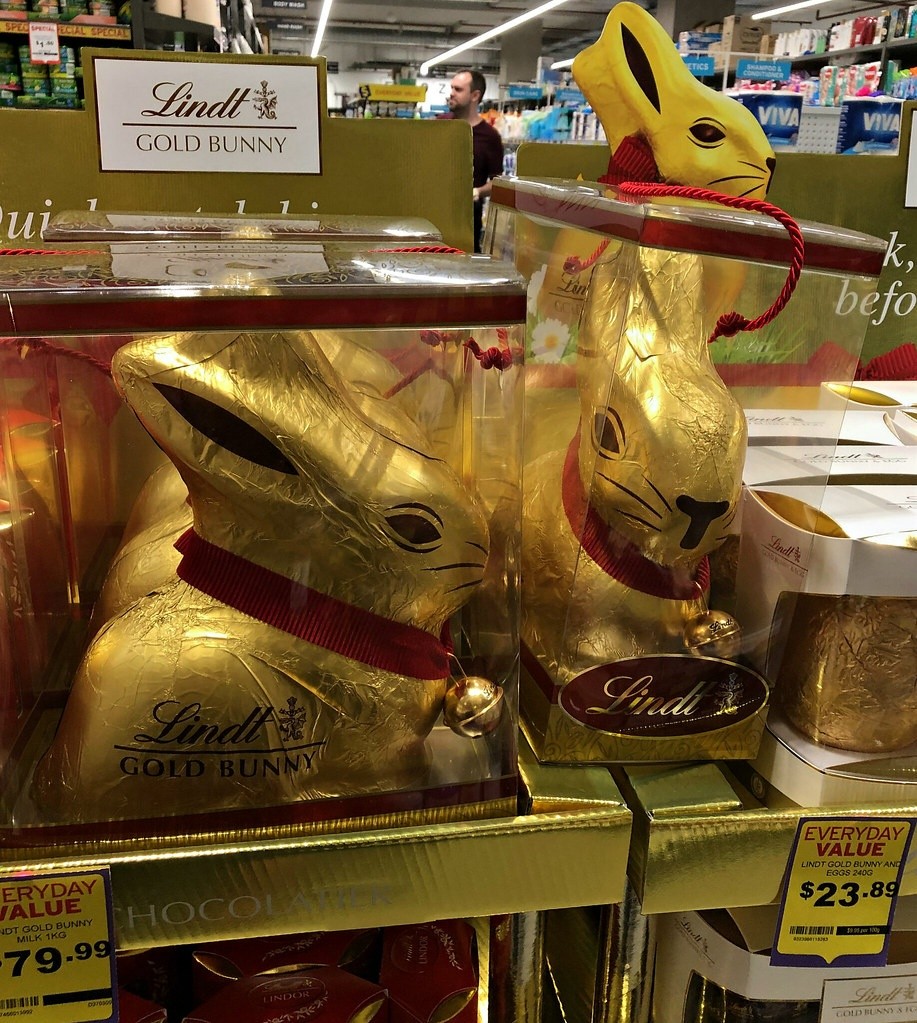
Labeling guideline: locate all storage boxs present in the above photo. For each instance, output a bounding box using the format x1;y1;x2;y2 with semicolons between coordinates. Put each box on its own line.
2;175;917;1023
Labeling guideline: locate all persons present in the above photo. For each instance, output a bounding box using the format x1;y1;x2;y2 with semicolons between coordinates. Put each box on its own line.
434;69;504;254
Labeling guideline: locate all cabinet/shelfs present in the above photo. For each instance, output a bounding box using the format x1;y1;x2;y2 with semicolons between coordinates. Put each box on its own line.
0;0;273;111
501;36;917;152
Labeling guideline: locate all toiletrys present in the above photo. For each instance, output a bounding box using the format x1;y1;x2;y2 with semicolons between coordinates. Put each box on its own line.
772;2;917;59
569;110;608;142
